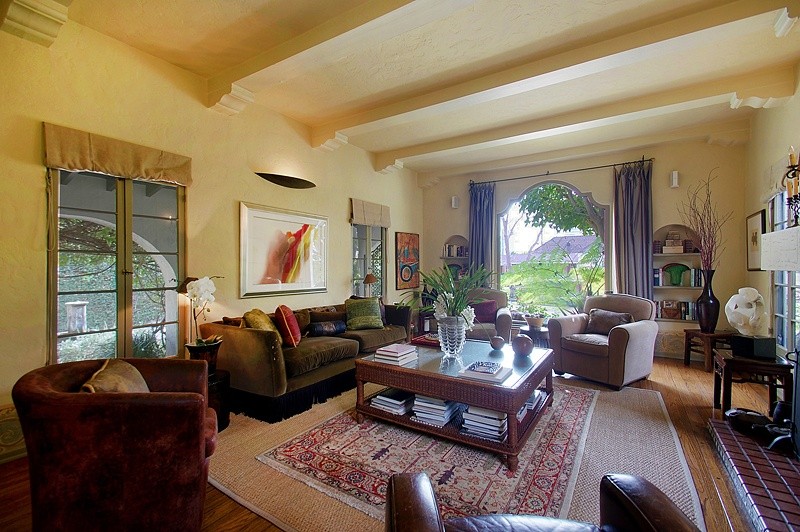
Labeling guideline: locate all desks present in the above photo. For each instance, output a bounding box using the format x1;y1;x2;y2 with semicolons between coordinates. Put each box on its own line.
683;329;735;372
712;349;795;419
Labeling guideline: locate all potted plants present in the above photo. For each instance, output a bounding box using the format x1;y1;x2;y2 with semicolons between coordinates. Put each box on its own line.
524;305;548;328
675;166;736;334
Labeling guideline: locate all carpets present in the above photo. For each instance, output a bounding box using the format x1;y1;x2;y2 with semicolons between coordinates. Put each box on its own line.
207;376;707;532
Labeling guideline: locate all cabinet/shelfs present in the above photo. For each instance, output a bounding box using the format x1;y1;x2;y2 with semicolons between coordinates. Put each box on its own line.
651;252;704;323
440;256;470;290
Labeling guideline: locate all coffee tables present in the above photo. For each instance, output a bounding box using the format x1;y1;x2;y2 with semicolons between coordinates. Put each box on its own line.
355;332;554;471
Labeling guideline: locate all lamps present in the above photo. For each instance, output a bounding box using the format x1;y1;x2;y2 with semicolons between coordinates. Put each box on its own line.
363;274;377;297
177;277;199;342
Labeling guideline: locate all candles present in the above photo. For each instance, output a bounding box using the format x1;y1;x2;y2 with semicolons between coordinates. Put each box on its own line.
789;144;797;166
786;179;792;198
792;177;798;195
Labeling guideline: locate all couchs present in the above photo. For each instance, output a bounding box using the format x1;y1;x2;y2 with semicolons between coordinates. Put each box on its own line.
12;359;218;532
199;304;412;398
385;472;702;532
548;293;658;391
430;288;512;344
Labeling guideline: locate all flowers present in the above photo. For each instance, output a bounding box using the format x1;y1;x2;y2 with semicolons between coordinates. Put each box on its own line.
395;262;500;330
186;274;225;342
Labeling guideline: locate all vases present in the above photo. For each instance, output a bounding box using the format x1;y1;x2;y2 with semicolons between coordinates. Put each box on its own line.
434;313;466;357
185;339;223;377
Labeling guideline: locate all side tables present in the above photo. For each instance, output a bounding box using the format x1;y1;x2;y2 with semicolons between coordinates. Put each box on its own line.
208;370;230;433
519;326;550;348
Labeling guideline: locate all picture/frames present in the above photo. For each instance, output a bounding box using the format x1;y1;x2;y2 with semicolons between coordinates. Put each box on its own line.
395;232;419;290
238;201;329;299
746;209;766;271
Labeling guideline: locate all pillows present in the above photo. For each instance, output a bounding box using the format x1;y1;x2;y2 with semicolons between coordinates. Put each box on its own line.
594;308;634;336
345;297;384;330
294;310;310;332
307;321;346;336
80;358;151;393
275;305;301;348
310;311;347;322
469;300;496;324
350;295;386;325
243;308;283;345
222;316;246;328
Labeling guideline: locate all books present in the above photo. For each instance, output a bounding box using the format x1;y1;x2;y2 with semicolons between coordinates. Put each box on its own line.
443;244;469;257
374;343;417;362
369;387;542;444
653;268;702;321
